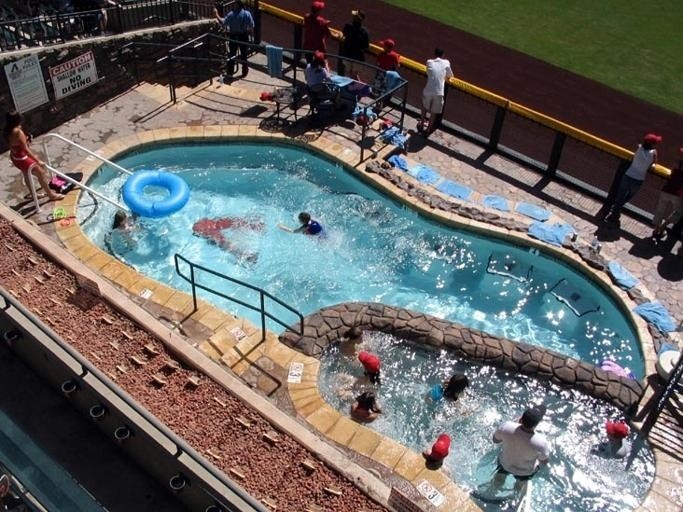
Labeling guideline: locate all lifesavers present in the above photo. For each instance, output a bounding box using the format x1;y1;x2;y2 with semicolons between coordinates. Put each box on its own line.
122;170;190;218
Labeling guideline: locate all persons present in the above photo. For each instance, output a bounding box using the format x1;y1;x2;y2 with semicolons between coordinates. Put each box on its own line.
276;211;322;237
605;131;663;224
337;352;383;389
304;48;336;98
649;147;681;241
111;210;139;233
350;393;382;422
590;420;629;460
490;403;550;482
422;432;451;465
211;0;254;85
423;371;468;416
376;35;400;112
7;107;65;201
335;8;369;78
190;216;266;264
302;0;340;74
415;45;454;141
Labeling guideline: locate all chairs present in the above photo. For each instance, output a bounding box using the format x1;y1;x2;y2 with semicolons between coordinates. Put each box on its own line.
273;82;340;128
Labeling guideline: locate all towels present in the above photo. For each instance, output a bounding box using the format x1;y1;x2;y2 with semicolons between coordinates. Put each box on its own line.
389;156;409;171
266;45;283;78
331;76;351;87
381;126;398;138
354;108;377;121
656;343;677;354
439;179;469;198
609;261;638;288
530;223;570;245
637;300;674;335
393;133;409;148
418;165;438;184
483;196;507;209
516;204;548;219
386;70;401;89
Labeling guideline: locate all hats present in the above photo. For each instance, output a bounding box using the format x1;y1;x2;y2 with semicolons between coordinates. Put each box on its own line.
315;16;330;28
312;1;326;11
351;9;367;21
644;134;662;145
522;405;546;425
358;352;380;370
236;0;247;10
315;51;326;62
380;39;395;48
432;434;450;457
607;423;628;437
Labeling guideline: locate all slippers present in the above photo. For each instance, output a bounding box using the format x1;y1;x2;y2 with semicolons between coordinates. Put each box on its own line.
49;193;64;200
